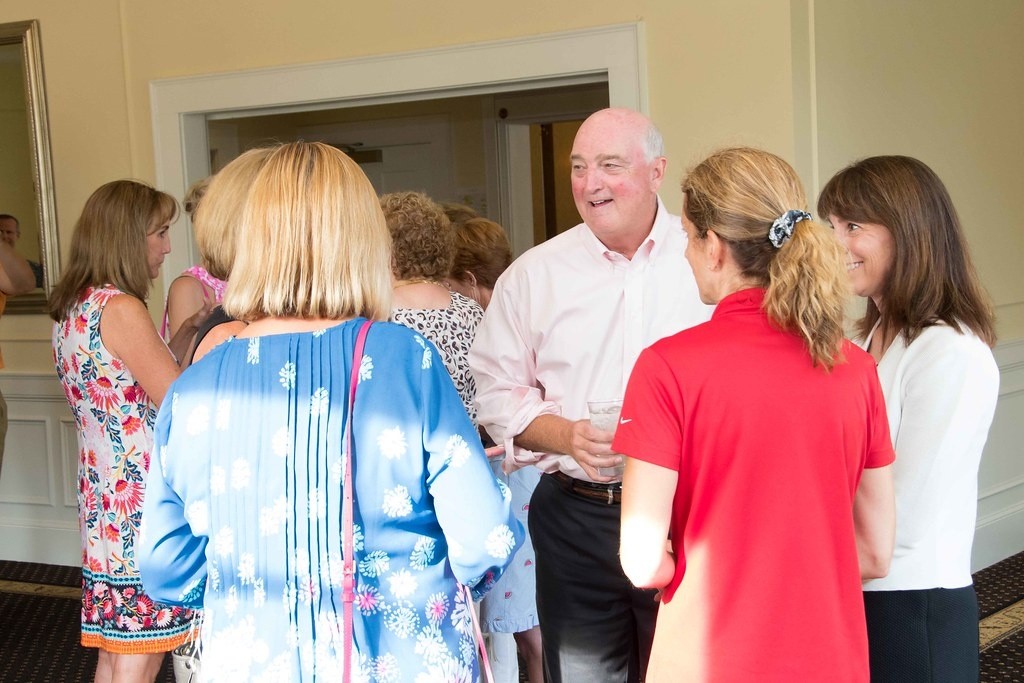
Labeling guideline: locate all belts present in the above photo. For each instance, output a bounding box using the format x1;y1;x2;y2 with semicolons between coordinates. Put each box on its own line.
549;471;622;503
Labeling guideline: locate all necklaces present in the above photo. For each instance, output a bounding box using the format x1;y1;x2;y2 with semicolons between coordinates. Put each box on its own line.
393;278;442;288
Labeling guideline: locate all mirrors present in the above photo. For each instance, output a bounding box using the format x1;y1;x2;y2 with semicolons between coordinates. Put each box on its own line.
0;13;66;320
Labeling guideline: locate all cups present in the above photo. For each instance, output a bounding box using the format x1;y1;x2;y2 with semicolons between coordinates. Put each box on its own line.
586;392;624;479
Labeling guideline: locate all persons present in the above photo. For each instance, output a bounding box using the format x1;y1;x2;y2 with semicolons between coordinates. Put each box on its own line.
0;238;37;473
611;148;895;683
816;153;1001;683
444;217;514;312
186;146;295;369
470;108;719;682
47;180;212;683
0;214;43;287
440;201;476;226
158;171;231;347
135;140;526;681
373;190;484;443
